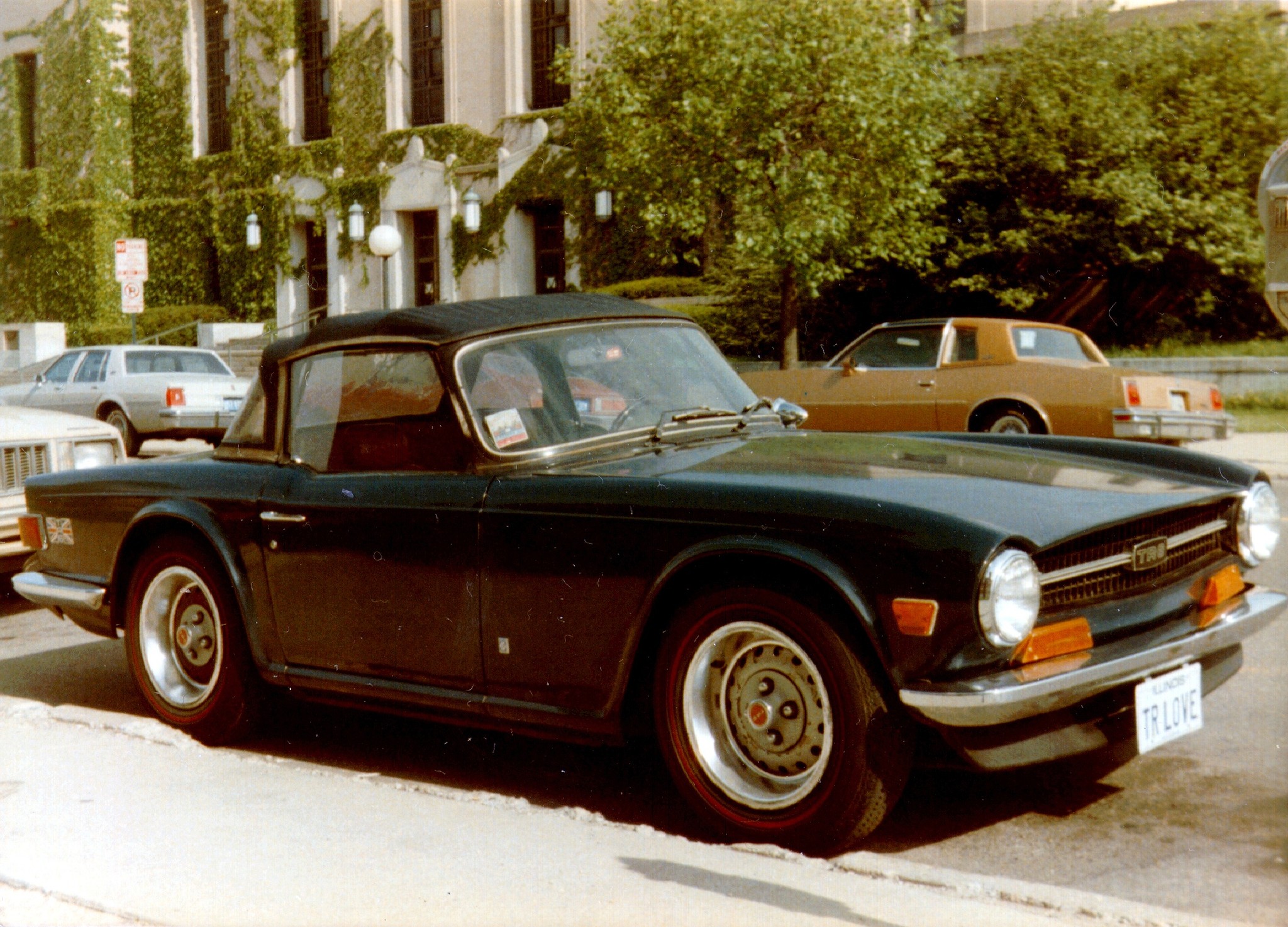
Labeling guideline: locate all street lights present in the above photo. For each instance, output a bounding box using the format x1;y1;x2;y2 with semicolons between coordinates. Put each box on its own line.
368;225;402;311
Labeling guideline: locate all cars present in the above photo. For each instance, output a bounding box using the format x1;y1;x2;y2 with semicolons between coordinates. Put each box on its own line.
297;352;626;438
0;343;255;458
8;293;1287;860
0;407;127;559
688;317;1231;450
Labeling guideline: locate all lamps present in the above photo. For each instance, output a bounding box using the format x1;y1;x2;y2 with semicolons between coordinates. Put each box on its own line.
463;184;482;233
245;209;262;252
347;199;366;243
593;188;613;224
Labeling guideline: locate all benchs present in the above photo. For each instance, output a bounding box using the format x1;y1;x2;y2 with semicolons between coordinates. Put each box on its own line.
292;406;446;474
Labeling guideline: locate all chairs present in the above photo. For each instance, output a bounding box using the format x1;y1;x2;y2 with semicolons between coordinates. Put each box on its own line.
355;415;468;474
